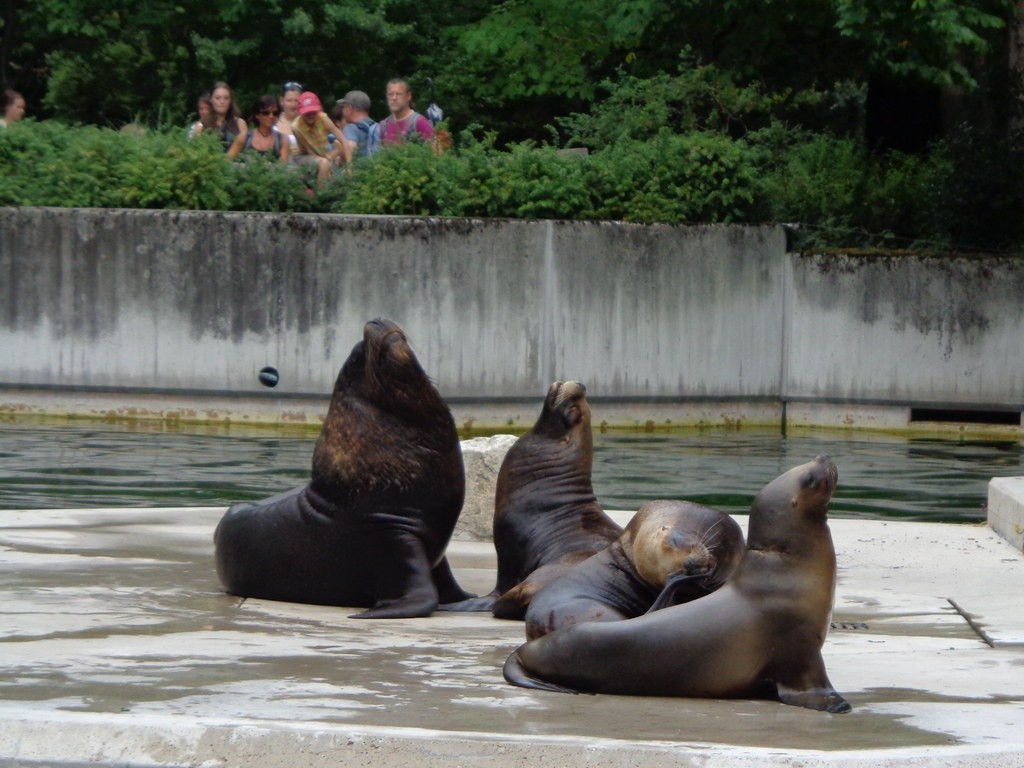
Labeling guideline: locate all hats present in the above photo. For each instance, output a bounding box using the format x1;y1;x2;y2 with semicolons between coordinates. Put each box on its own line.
296;91;322;115
336;91;371;112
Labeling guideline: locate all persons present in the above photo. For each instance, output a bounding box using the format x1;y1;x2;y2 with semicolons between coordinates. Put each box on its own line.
188;79;434;187
0;90;26;127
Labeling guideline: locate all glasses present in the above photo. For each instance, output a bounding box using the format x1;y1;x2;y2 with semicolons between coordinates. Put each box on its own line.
258;110;280;116
283;82;303;91
